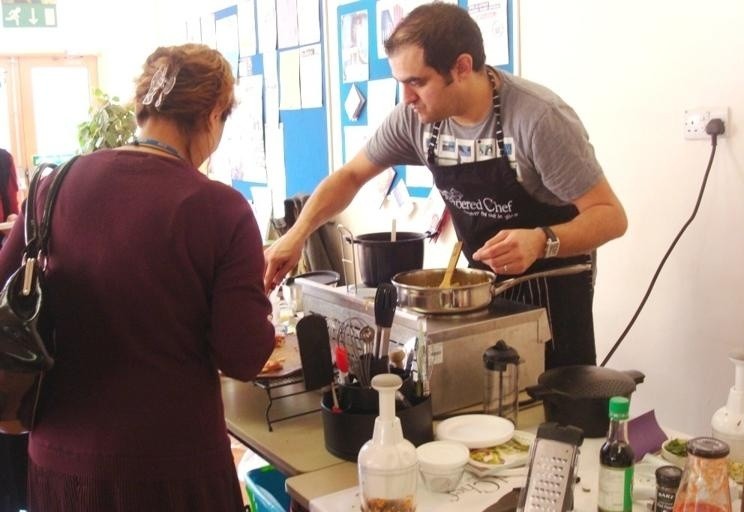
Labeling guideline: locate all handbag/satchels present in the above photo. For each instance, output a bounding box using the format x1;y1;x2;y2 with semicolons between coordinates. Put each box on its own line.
1;155;80;434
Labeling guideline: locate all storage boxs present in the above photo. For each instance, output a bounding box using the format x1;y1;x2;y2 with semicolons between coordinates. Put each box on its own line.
242;465;295;512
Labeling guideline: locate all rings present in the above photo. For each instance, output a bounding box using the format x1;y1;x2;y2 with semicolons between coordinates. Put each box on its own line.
504;265;508;272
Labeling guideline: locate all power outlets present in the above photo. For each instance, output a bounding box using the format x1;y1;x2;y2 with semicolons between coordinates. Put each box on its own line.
682;107;707;142
707;106;730;140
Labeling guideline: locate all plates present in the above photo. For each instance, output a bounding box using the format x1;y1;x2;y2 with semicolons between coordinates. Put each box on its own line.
436;412;533;470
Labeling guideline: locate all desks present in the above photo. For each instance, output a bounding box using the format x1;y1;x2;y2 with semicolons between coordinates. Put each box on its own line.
218;373;532;475
283;405;544;512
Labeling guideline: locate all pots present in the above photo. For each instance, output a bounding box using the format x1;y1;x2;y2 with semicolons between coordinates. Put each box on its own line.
527;365;645;438
346;231;593;315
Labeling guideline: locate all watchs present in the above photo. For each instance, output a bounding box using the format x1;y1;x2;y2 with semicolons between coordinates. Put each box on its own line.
541;226;561;260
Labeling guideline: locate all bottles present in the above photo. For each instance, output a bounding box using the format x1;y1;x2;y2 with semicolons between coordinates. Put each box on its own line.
358;372;417;511
598;397;732;512
484;339;521;418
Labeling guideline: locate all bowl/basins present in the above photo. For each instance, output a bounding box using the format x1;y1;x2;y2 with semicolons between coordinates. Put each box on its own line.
416;439;471;489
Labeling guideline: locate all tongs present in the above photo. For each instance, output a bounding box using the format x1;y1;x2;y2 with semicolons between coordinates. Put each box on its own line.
371;280;397;364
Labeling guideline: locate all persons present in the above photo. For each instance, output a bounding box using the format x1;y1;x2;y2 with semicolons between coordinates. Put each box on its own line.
0;148;19;249
0;42;277;511
264;2;628;372
